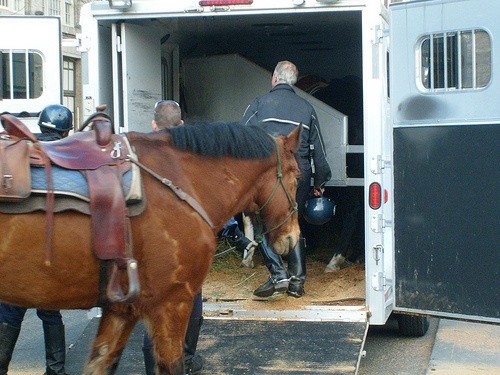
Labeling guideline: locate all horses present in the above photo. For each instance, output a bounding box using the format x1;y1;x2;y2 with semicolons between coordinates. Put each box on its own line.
0;120;308;375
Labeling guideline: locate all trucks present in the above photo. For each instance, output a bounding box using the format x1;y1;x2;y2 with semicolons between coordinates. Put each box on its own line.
0;0;500;375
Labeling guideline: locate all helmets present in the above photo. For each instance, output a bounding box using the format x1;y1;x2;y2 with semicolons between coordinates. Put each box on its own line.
302;197;336;224
38;106;73;131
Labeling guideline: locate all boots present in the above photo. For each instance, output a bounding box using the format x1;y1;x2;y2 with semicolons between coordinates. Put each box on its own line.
287;238;307;297
183;293;203;374
43;325;65;375
0;323;21;375
252;236;288;298
143;346;157;375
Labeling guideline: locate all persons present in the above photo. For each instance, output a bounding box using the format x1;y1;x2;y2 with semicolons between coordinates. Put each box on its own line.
1;105;75;375
242;60;331;298
140;99;204;375
